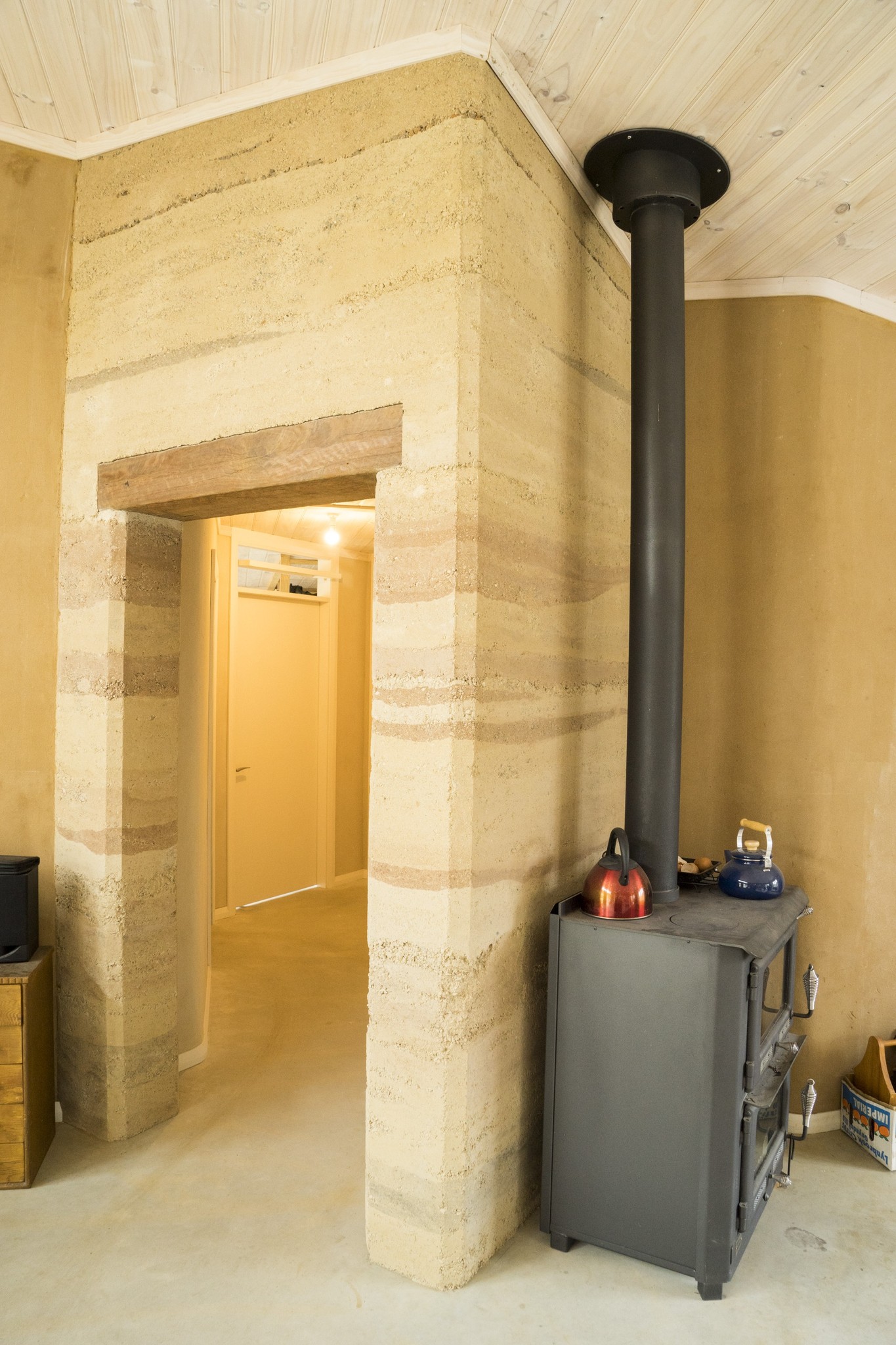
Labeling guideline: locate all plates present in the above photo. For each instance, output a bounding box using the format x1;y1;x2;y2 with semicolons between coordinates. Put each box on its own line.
677;857;720;881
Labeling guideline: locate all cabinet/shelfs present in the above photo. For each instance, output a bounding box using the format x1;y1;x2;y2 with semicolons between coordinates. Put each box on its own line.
0;947;56;1190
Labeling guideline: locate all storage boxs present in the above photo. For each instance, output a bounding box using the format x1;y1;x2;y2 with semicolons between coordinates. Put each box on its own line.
0;854;40;964
839;1073;896;1171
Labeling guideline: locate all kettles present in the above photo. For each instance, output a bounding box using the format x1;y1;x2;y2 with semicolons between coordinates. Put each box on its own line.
719;818;785;899
581;828;651;919
